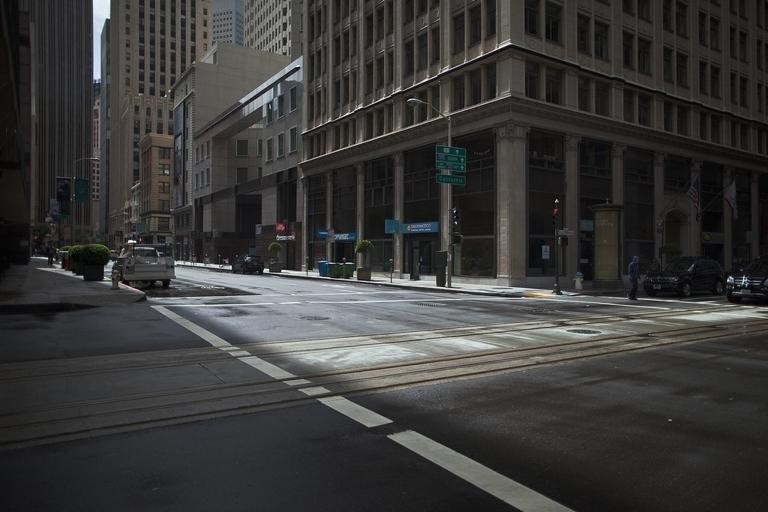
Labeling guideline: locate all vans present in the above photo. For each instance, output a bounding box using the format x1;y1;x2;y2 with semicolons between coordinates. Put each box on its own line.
109;249;118;259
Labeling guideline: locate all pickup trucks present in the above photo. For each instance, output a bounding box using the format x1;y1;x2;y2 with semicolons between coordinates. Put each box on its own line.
112;245;176;289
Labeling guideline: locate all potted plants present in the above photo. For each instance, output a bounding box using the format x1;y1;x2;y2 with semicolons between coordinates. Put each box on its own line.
353;238;375;280
267;242;283;272
59;243;111;281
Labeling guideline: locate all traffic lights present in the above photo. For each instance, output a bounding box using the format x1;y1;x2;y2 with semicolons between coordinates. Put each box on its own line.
448;207;458;227
550;208;559;228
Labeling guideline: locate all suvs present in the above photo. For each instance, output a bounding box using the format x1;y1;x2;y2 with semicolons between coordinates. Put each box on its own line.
721;255;767;306
641;255;725;296
230;253;265;275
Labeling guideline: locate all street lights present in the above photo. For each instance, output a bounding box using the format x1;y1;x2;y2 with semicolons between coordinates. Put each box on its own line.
70;156;101;246
406;97;453;289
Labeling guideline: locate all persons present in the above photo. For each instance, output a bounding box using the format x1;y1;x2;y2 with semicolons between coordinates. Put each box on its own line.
626;254;642;301
44;242;54;266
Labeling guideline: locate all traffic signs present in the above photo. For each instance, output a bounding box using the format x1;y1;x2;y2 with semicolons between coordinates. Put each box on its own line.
435;144;466;157
435;160;466;172
435;153;466;164
434;173;466;185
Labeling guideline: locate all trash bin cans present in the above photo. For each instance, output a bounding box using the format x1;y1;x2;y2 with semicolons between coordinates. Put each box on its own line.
317;261;356;278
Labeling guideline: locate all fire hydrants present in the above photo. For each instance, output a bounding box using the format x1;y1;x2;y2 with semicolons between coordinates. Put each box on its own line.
109;268;121;291
